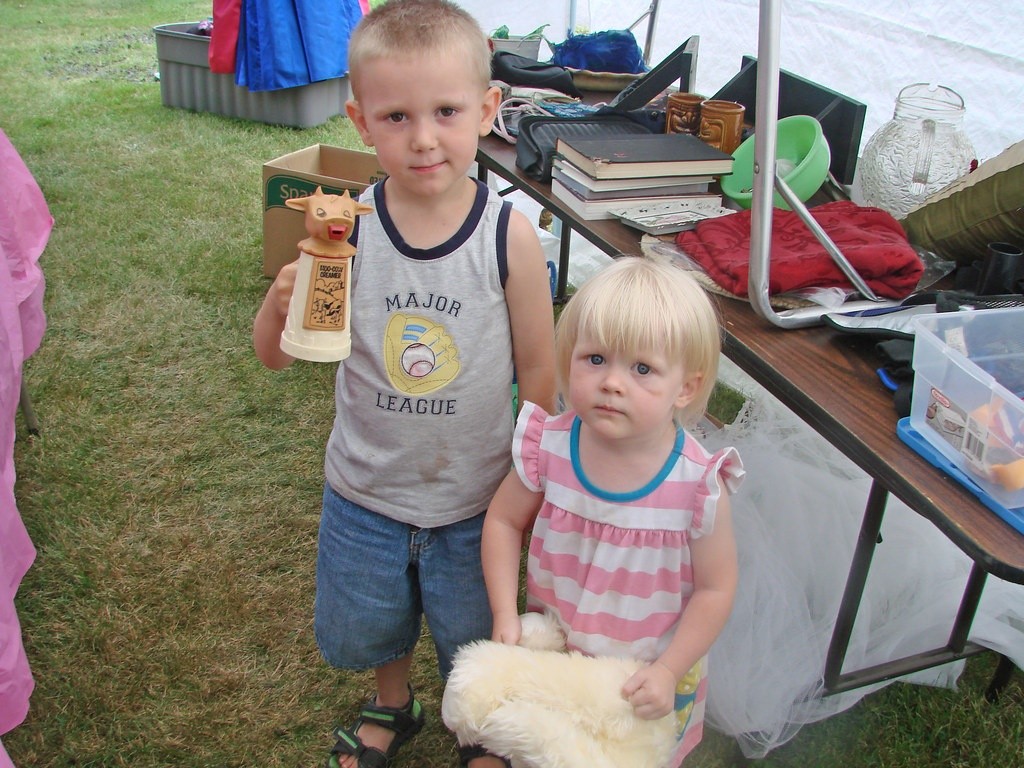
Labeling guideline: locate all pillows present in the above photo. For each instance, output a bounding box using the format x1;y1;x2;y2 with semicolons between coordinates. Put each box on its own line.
897;140;1024;265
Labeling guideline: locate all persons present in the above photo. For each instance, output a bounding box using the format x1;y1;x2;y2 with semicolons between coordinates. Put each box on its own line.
479;251;748;768
249;0;557;768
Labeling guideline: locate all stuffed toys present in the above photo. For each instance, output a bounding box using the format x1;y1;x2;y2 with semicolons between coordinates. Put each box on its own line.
440;612;680;768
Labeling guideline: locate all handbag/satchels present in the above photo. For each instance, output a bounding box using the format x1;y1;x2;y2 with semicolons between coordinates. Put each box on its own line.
515;115;654;184
490;50;585;99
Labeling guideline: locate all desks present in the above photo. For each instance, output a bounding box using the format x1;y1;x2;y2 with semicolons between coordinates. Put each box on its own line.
477;134;1024;768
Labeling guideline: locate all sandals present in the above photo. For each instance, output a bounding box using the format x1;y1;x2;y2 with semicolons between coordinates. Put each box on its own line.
327;681;424;768
456;738;513;768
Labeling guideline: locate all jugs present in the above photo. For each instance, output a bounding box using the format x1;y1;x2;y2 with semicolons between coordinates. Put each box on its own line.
860;82;977;217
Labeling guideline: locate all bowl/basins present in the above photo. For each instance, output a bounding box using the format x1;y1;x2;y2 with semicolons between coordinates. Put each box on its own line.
721;115;830;209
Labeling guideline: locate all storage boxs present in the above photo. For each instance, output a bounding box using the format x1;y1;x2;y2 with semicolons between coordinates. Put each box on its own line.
260;143;388;278
896;304;1024;537
151;21;351;128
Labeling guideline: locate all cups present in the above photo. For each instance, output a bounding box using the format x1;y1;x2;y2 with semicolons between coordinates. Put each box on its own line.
666;93;706;139
975;242;1022;294
699;99;745;156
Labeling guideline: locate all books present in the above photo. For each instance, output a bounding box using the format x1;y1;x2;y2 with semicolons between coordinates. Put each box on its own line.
550;132;735;220
